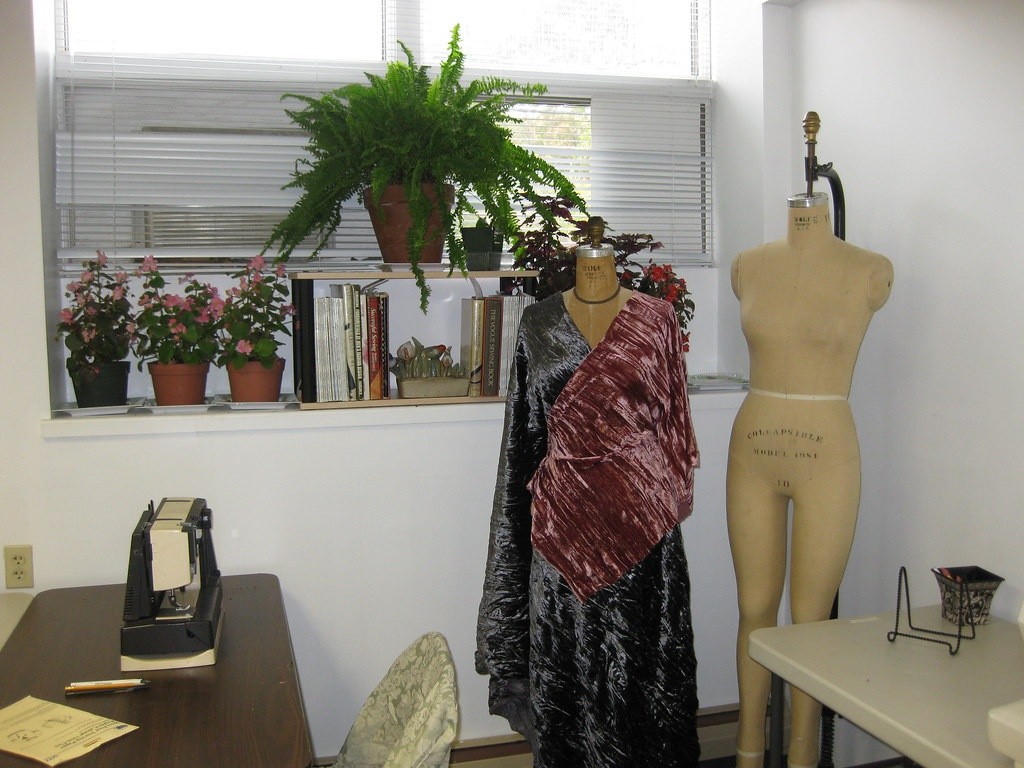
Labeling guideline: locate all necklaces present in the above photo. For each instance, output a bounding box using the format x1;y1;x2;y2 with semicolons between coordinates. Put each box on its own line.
573;283;621;304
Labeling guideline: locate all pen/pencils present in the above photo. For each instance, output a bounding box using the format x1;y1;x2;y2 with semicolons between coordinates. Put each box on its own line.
64;678;152;696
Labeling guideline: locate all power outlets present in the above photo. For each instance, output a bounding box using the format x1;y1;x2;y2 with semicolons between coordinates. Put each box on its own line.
4;545;33;588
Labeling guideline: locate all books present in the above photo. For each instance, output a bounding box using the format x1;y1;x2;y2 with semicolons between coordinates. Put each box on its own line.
290;278;390;403
460;294;535;398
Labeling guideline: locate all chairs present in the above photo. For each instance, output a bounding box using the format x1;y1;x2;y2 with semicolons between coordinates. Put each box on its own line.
327;631;458;768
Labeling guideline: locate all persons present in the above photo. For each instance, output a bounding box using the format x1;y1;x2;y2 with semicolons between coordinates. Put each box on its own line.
726;192;895;768
474;244;701;768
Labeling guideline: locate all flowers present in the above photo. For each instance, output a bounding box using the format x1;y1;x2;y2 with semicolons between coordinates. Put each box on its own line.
54;251;296;368
620;261;695;353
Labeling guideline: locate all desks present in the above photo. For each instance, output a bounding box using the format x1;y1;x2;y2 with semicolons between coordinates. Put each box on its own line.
0;572;315;768
748;603;1024;767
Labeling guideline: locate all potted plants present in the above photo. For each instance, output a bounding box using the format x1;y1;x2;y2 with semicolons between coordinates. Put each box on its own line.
388;336;470;398
460;217;502;270
260;22;614;315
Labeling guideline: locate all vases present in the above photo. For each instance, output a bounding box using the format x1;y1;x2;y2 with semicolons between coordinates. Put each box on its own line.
53;362;147;417
213;359;301;410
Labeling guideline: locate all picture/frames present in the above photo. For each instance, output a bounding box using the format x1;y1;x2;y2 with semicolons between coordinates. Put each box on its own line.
134;361;222;413
